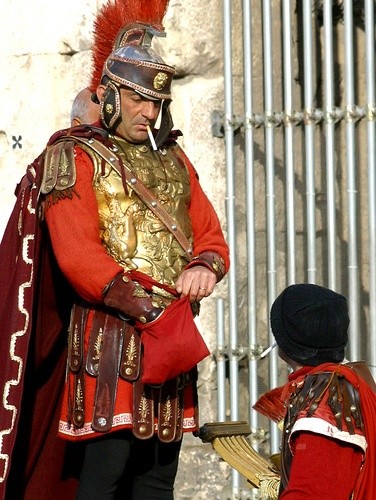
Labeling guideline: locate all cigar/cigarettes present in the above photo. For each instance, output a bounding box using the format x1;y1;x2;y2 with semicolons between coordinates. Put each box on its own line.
146;125;159;151
260;341;279;359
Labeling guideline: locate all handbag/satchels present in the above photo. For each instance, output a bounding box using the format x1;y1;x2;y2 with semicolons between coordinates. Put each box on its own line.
121;269;211;388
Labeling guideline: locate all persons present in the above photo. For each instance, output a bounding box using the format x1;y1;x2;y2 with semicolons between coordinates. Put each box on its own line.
256;284;376;500
38;44;230;499
71;86;102;127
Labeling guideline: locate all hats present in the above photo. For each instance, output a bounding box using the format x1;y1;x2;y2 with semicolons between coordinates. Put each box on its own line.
87;0;178;152
270;283;350;368
